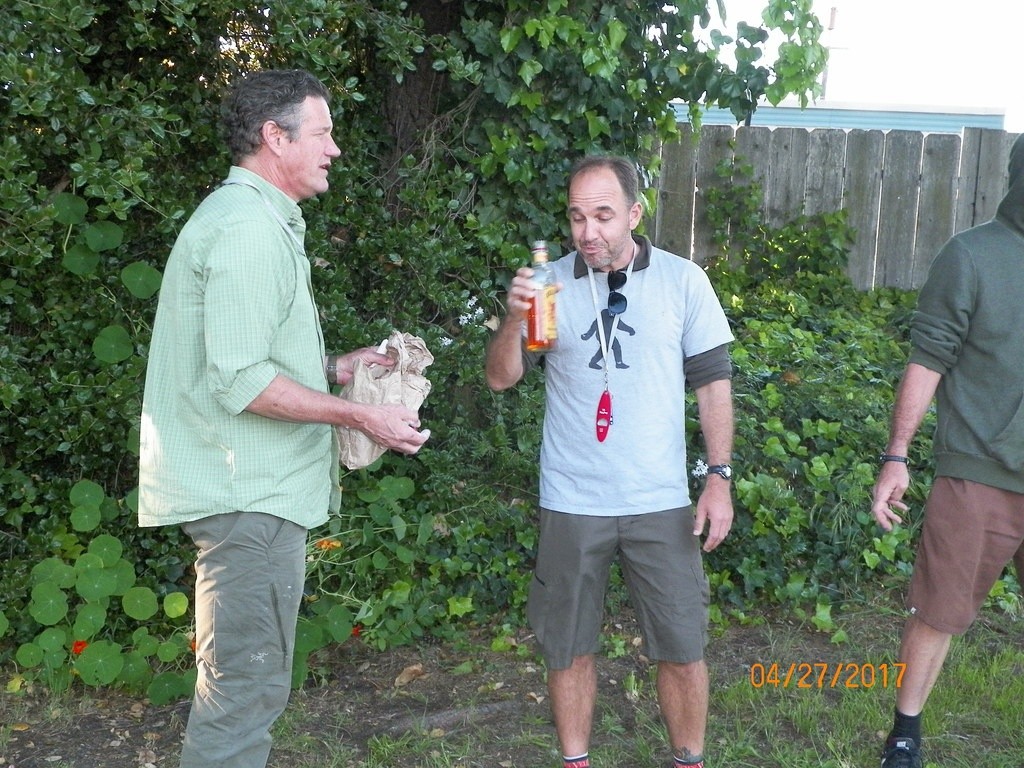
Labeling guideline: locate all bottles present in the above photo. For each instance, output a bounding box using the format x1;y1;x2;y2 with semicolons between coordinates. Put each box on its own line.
518;240;558;351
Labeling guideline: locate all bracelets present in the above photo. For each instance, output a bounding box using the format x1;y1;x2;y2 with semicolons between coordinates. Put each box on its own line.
879;452;908;466
327;356;338;385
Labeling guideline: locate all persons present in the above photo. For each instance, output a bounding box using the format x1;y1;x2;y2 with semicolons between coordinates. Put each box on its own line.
486;154;735;768
138;69;432;768
870;135;1024;768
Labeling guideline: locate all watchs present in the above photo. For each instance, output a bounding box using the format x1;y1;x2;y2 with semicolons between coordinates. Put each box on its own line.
707;464;733;480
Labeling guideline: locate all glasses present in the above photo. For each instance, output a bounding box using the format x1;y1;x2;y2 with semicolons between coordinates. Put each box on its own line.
608;270;627;316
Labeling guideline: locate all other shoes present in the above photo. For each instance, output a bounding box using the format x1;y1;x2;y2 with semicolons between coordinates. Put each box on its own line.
880;736;924;768
